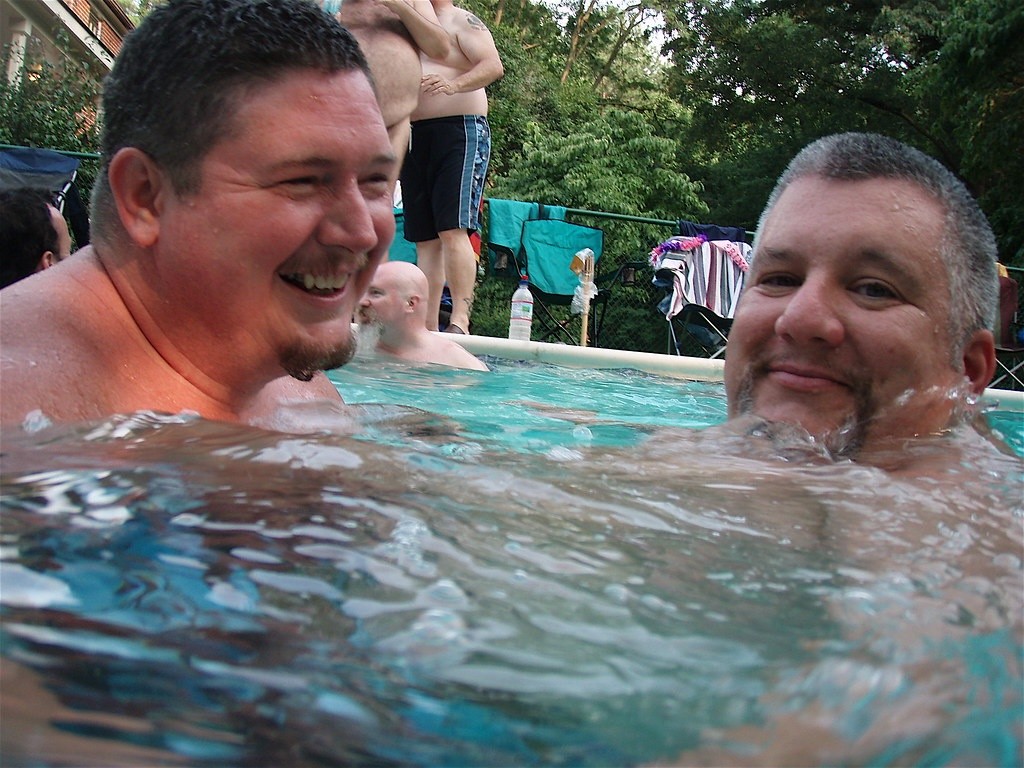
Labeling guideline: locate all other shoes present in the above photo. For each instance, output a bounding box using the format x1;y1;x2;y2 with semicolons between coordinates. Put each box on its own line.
444;324;465;334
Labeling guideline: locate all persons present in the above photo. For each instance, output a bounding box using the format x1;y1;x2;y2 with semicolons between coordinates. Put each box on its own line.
356;261;490;372
308;0;450;191
400;0;503;337
0;0;400;440
0;186;72;290
721;129;1024;626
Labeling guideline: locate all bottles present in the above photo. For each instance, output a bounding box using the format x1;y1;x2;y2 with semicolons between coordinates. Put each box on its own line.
508;276;533;341
